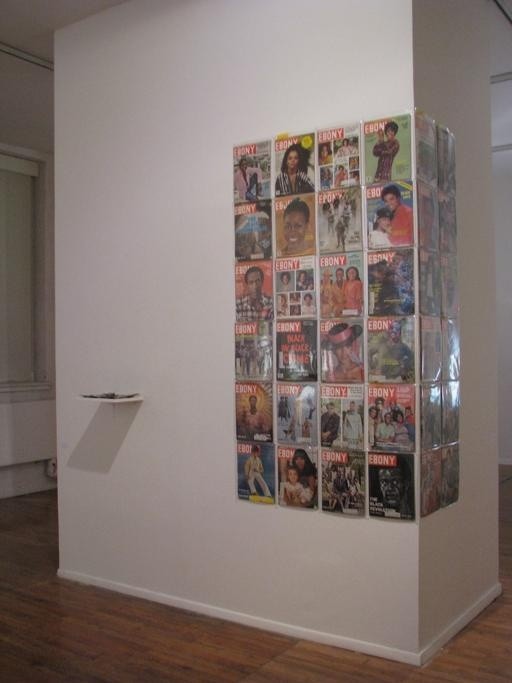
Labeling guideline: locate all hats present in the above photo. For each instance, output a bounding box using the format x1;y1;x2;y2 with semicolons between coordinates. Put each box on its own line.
372;208;392;224
320;322;362;351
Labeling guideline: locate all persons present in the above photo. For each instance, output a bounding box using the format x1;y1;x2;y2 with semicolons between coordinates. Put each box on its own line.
235;265;273;319
279;197;316;258
233;158;265;201
278;391;414;450
321;189;360;253
241;393;269;440
381;184;413;245
319;137;359;188
342;266;363;315
322;320;363;382
331;267;346;316
278;269;316;316
237;339;273;378
370;120;402;179
243;443;272;498
368;207;394;247
282;447;414;519
274;142;315;196
321;268;334;317
